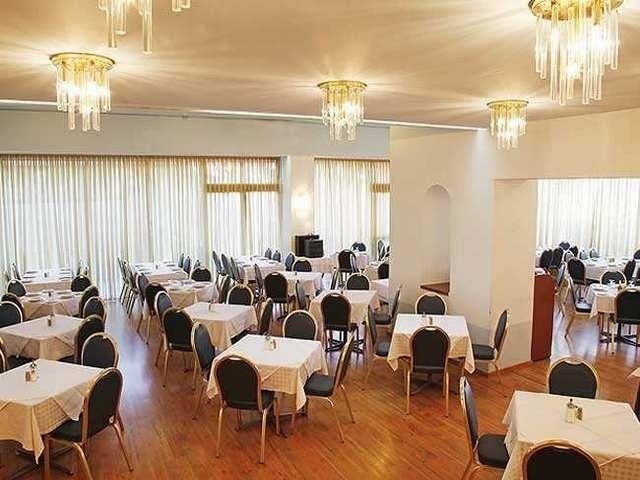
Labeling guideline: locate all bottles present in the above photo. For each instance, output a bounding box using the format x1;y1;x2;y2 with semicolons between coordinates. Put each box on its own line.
25;360;39;381
264;334;278;350
608;280;635;292
206;300;217;314
48;310;55;327
566;398;583;423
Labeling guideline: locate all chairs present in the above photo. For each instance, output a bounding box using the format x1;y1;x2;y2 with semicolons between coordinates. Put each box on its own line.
118;242;511;464
460;376;528;480
0;256;133;480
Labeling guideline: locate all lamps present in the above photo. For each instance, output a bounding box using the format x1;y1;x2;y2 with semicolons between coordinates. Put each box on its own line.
315;80;369;145
486;99;530;151
526;0;625;107
47;51;115;134
98;0;190;54
